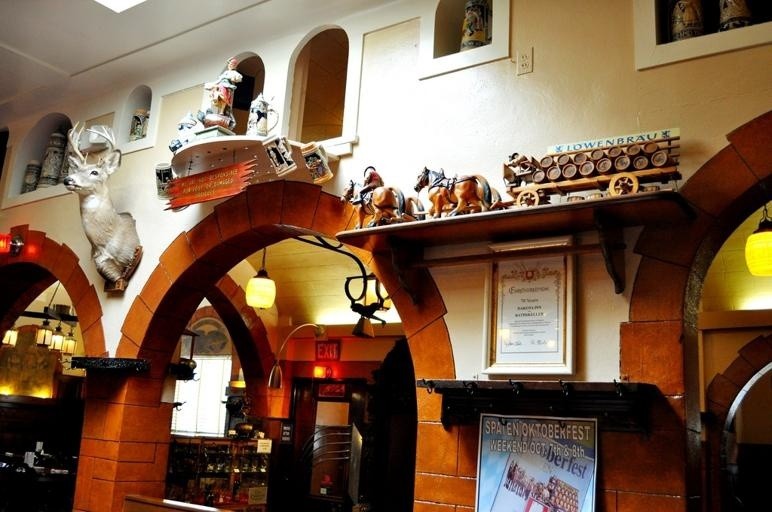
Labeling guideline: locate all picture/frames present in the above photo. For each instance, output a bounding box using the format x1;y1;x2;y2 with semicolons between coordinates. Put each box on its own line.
480;234;578;377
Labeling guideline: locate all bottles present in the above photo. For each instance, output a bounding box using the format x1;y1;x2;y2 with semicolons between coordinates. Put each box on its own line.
22;132;70;191
233;478;240;501
130;108;150;139
674;0;751;38
462;1;485;49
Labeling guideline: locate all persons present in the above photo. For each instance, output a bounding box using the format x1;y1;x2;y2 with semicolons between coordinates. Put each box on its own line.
206;59;243;115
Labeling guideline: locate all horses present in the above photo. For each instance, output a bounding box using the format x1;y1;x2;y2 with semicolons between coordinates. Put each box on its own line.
339;166;501;229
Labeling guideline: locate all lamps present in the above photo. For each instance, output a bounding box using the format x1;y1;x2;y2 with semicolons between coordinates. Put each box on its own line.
245;246;277;310
35;318;78;356
2;322;20;349
268;322;326;388
744;204;772;276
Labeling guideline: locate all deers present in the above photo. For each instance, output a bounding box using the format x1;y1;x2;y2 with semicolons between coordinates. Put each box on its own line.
58;121;140;286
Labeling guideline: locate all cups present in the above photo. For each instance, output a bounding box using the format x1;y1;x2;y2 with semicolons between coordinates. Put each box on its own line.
246;101;278;139
262;137;334;186
155;163;173;200
